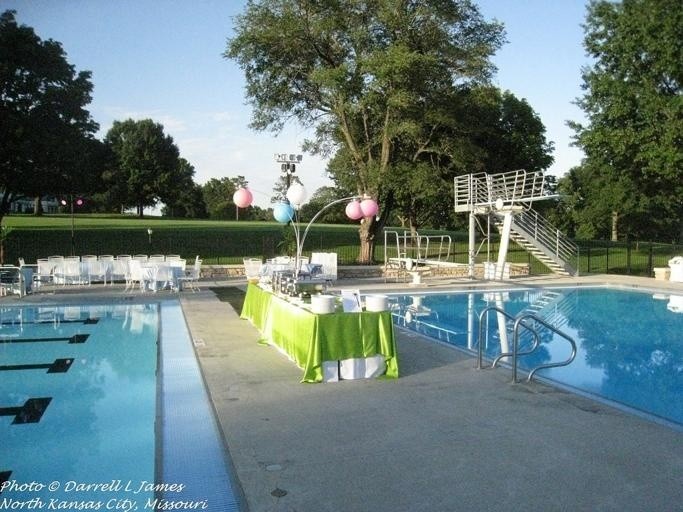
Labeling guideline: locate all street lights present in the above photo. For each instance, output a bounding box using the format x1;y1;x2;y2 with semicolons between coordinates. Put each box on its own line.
273;154;303;227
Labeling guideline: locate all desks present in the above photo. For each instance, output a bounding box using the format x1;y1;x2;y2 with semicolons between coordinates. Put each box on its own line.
248;279;392;380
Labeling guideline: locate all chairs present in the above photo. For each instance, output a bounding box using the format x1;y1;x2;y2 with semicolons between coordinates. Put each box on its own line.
243;252;338;281
0;252;204;299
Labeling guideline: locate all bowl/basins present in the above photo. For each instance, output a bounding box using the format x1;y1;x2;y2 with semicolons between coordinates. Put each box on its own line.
366;293;388;311
312;294;336;314
